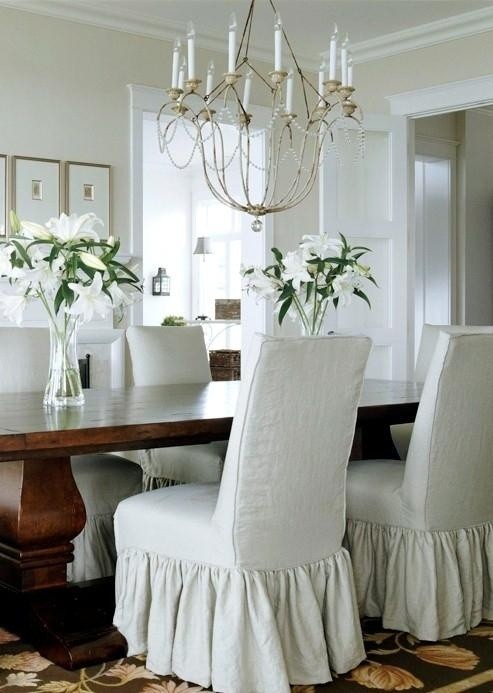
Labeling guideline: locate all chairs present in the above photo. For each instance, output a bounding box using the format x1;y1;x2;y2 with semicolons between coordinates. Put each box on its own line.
340;324;492;643
0;327;141;589
207;347;239;380
412;323;443;379
214;297;239;319
108;334;382;692
120;322;229;492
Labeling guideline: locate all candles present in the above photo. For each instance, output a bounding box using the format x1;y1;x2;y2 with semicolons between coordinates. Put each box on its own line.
148;0;370;234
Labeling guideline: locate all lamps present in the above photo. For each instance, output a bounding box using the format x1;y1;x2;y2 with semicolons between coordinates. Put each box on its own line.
192;232;215;264
152;263;171;299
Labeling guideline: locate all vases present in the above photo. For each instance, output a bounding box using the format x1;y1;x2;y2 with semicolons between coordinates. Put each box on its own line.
43;318;84;409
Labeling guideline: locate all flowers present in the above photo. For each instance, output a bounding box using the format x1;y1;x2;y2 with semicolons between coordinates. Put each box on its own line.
235;225;380;337
3;205;146;331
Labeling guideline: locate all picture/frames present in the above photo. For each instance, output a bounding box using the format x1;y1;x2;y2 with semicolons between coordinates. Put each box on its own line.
63;160;112;238
11;153;63;240
0;153;7;236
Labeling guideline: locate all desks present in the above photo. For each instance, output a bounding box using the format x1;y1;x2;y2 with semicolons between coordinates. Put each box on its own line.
181;316;241;350
0;375;426;672
